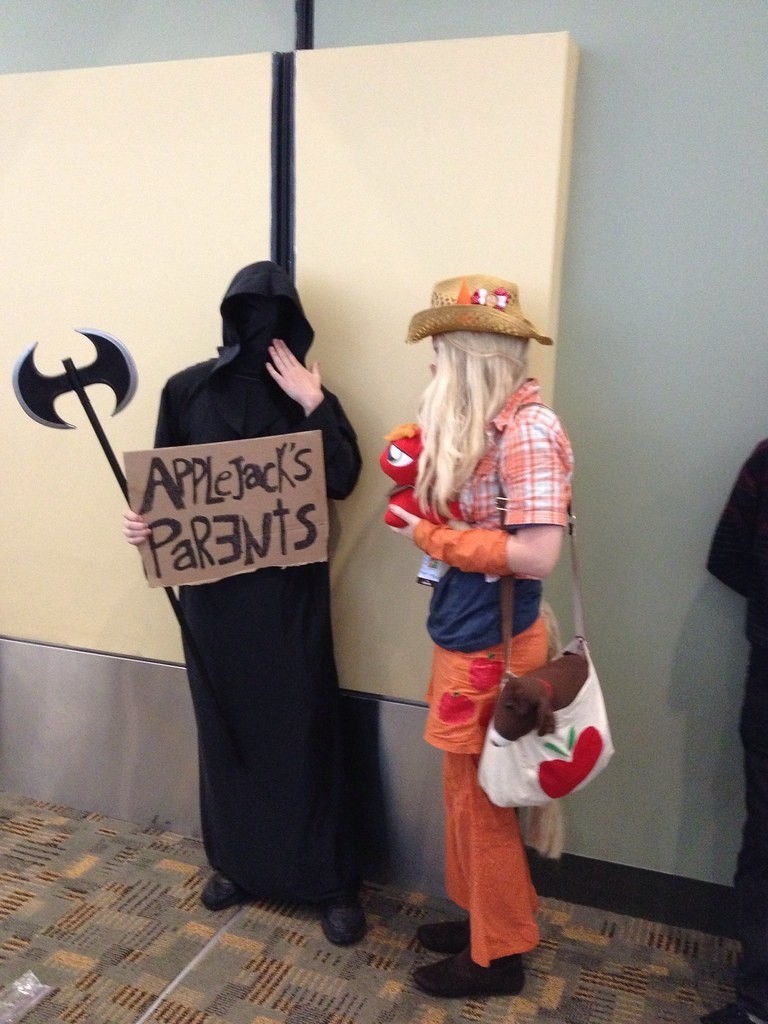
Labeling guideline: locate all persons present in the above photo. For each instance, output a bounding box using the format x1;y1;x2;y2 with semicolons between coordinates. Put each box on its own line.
123;260;394;946
699;437;768;1024
385;272;576;998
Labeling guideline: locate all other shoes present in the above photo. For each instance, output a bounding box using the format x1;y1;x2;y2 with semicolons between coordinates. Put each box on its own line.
698;1000;768;1024
321;884;367;944
200;873;250;911
418;915;472;955
410;950;524;1001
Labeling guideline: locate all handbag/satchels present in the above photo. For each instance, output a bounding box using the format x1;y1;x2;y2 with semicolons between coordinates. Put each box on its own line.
476;639;615;807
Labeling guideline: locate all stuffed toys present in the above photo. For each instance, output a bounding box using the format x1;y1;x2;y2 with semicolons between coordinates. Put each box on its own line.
380;423;475;528
488;653;589;747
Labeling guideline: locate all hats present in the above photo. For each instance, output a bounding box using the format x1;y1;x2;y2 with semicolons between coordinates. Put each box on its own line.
407;274;553;346
